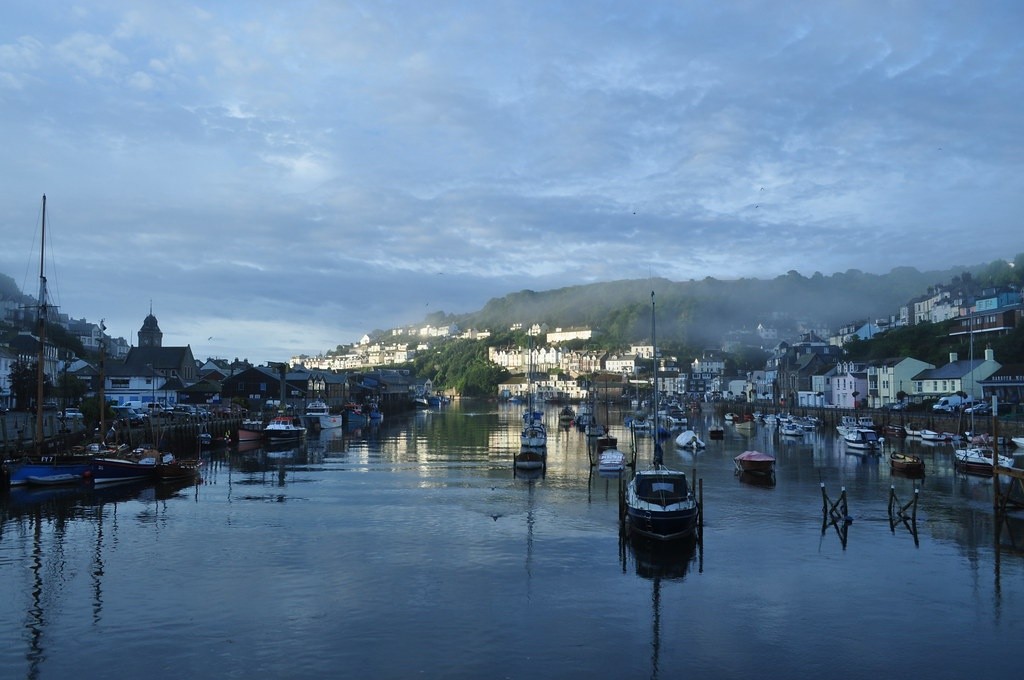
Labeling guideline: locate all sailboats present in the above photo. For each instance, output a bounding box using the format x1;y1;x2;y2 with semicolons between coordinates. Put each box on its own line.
706;383;725;435
516;333;548;470
950;308;1016;472
576;345;619;447
625;289;699;535
1;194;210;489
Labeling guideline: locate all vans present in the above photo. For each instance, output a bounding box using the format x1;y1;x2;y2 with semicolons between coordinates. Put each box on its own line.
932;396;970;412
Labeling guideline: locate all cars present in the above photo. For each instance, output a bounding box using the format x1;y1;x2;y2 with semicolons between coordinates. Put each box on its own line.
265;400;293;411
57;400;250;425
894;404;909;410
953;400;1012;416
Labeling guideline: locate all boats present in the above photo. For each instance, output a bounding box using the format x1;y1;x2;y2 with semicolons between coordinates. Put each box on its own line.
725;413;739;422
196;414;307;443
628;392;698;433
297;396;384;430
1011;437;1024;447
559;404;575;421
411;389;450;407
734;450;776;473
889;451;925;472
675;429;705;449
508;397;518;402
735;411;952;450
592;466;623;479
598;450;625;470
